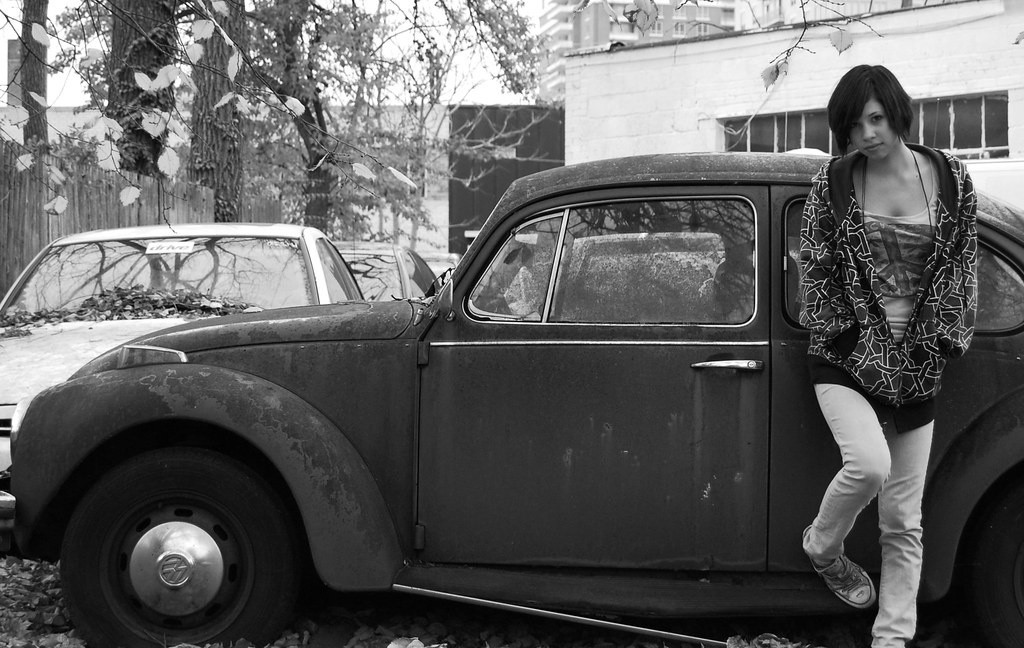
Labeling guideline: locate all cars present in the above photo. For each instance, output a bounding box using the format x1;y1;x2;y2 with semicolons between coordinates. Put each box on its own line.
0;224;366;533
506;233;723;325
7;151;1024;647
332;239;461;304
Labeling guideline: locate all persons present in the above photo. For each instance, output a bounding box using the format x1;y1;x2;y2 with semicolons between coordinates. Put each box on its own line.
796;64;977;648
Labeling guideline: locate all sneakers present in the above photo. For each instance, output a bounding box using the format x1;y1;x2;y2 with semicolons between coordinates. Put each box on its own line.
802;525;876;608
